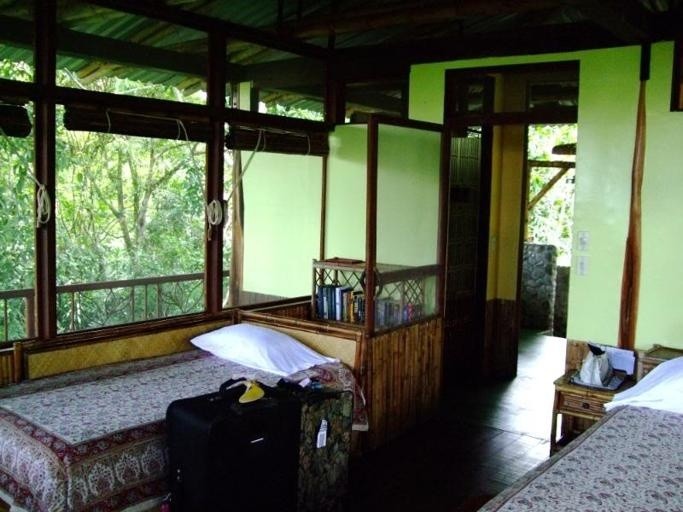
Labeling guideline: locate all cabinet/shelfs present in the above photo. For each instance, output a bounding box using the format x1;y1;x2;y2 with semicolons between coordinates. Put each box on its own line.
308;257;427;331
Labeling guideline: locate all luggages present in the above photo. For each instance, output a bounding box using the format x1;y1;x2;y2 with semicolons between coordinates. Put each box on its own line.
297;380;356;511
161;376;301;512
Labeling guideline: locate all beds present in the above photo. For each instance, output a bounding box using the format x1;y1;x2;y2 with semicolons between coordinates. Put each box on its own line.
478;354;682;512
0;350;371;511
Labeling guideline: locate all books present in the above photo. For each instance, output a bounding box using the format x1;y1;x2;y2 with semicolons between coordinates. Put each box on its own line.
317;284;423;328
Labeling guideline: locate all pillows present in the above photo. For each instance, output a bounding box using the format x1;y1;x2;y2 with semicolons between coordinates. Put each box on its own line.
189;322;341;378
603;356;683;419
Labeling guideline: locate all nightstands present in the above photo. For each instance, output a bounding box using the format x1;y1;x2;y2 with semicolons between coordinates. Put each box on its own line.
550;366;636;458
